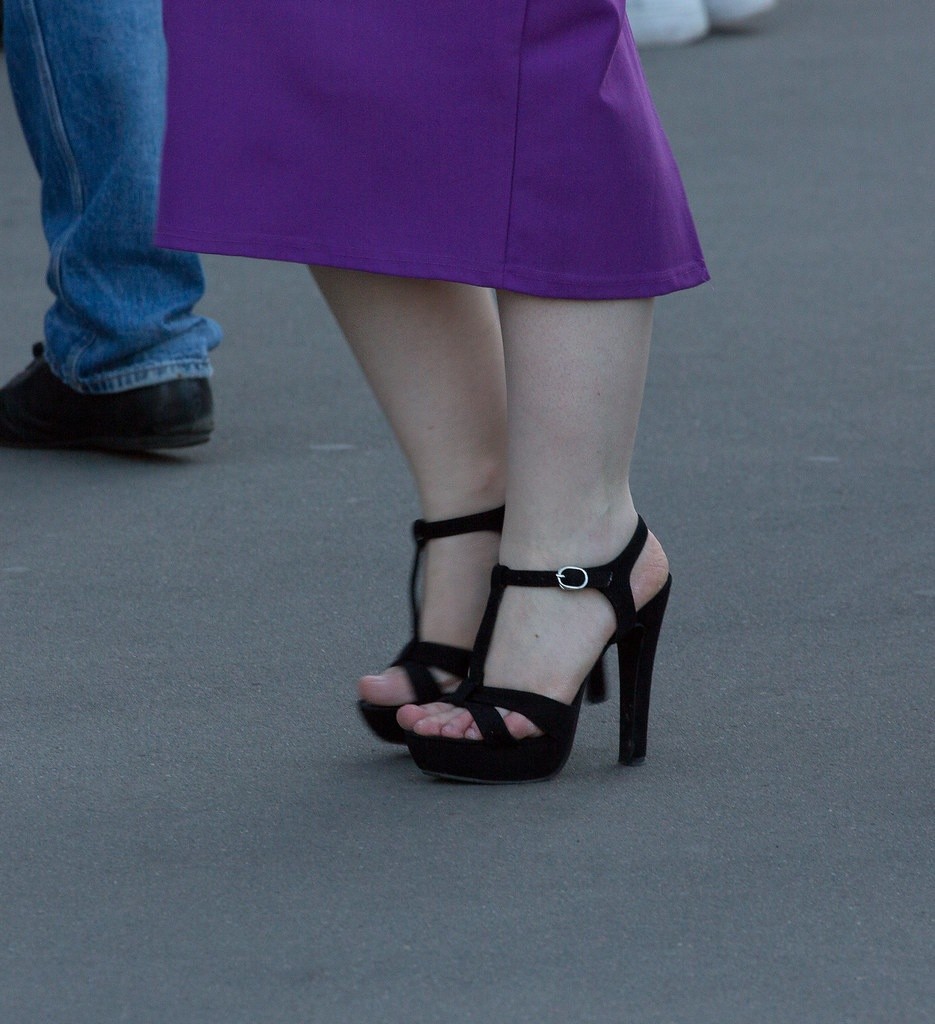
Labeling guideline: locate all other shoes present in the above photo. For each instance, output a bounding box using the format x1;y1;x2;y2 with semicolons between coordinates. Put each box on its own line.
624;0;772;48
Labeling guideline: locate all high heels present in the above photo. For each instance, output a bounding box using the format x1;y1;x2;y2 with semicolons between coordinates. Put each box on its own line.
359;503;608;747
402;513;672;783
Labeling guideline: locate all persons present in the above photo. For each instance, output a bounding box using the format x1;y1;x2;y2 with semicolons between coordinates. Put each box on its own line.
624;0;777;47
151;0;710;783
0;0;223;453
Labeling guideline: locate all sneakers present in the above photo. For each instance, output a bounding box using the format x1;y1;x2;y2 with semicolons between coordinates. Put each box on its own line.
0;343;213;448
